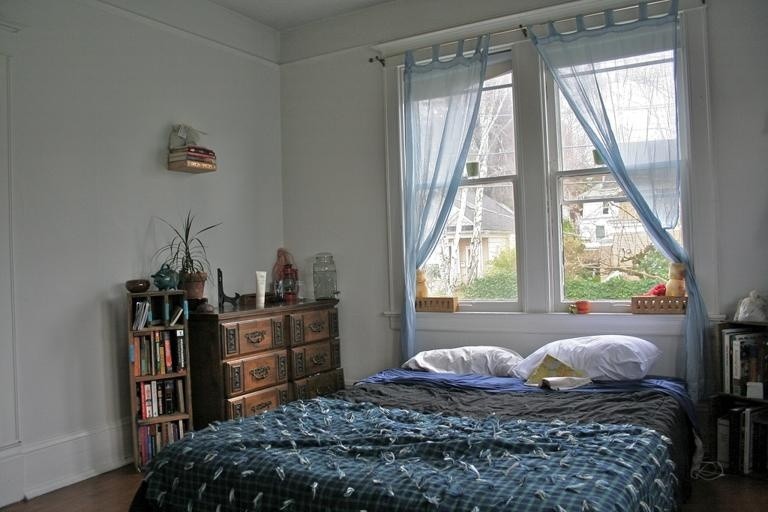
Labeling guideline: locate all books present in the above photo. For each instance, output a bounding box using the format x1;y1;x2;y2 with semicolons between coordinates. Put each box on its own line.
713;327;768;474
129;299;189;471
168;144;217;175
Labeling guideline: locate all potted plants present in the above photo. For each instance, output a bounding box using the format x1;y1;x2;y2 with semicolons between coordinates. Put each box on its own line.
147;208;224;299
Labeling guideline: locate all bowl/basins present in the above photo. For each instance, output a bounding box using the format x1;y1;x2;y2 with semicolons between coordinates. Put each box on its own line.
125;280;150;292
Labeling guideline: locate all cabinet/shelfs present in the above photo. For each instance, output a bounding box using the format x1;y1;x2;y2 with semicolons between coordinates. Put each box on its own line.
127;291;193;475
192;298;346;430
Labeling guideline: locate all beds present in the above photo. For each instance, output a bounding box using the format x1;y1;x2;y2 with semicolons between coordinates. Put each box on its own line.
137;362;688;512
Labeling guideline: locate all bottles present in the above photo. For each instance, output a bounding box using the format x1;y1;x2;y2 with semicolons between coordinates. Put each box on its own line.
313;253;336;301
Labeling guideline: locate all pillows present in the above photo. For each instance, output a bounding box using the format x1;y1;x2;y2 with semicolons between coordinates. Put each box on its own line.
398;333;664;382
